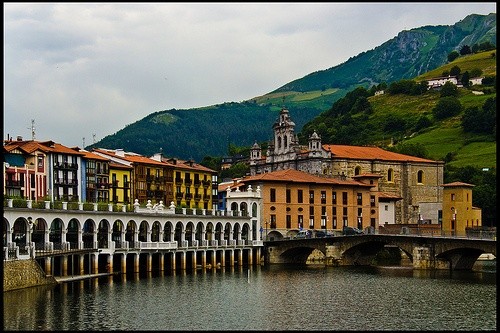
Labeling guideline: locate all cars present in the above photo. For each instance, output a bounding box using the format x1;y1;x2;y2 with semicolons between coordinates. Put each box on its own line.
315;230;334;237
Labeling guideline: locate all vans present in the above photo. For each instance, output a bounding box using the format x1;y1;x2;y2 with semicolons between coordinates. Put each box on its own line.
293;231;308;240
343;227;364;235
